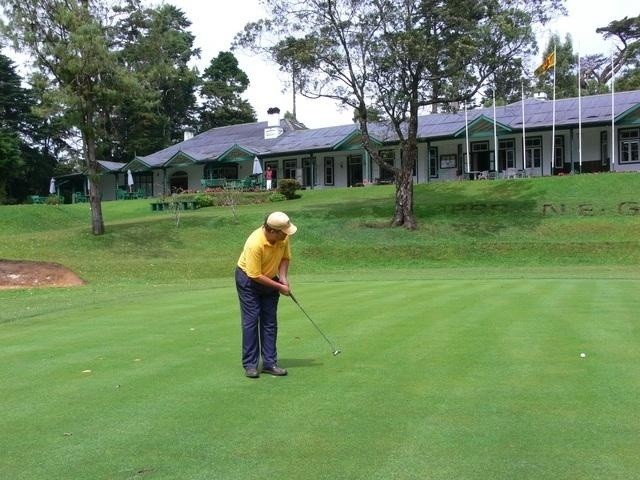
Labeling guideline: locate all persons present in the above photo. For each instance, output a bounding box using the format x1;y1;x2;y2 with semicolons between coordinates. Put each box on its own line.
234;210;298;379
263;166;273;192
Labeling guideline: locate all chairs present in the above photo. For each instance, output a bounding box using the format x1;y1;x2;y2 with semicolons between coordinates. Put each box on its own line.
114;187;143;199
24;192;103;206
467;168;530;179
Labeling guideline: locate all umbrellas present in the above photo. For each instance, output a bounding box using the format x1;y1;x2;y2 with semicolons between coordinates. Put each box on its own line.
126;169;136;193
252;156;263;182
49;177;56;193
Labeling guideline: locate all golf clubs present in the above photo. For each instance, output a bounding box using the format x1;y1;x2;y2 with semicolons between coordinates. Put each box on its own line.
285;286;341;356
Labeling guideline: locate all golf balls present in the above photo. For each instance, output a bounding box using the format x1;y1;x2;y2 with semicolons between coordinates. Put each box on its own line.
580;352;587;359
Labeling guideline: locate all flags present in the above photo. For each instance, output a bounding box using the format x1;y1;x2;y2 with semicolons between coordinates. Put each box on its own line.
579;54;599;76
533;51;555;78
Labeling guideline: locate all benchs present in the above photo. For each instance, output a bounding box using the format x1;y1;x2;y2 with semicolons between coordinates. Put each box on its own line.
199;176;257;192
150;200;201;212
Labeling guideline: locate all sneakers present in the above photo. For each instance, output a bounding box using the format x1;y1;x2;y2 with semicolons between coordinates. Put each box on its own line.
262;366;287;376
245;368;259;377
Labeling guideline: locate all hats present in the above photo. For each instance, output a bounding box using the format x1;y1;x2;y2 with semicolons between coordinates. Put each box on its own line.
268;212;297;235
